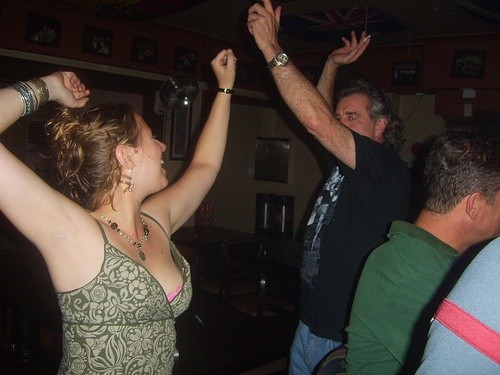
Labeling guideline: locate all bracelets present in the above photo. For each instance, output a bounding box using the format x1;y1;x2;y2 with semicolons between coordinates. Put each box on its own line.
8;77;49;118
217;88;234;94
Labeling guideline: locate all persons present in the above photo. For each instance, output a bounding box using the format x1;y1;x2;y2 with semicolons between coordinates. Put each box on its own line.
0;48;237;375
248;0;412;375
345;131;500;375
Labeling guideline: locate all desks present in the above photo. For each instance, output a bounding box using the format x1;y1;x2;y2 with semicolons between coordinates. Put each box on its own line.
170;224;303;375
0;250;63;375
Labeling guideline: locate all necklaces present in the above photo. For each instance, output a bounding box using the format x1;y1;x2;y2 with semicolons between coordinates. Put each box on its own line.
96;211;150;261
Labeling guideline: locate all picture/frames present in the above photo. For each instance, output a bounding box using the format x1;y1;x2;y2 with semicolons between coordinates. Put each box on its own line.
392;61;422;85
450;49;487;81
131;36;158;66
174;45;198;73
82;24;114;56
25;12;61;47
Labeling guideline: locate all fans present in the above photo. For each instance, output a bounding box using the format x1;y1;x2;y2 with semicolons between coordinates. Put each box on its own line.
154;73;199;116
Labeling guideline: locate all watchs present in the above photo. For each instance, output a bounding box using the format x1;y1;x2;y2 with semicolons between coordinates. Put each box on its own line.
265;50;292;72
